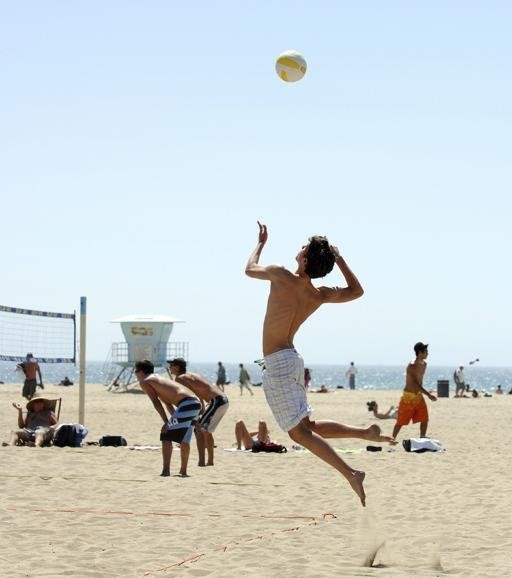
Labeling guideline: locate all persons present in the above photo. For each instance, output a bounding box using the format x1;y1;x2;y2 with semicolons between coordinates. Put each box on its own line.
391;341;437;444
495;385;503;394
134;360;287;477
1;398;58;447
454;366;466;397
304;368;311;388
345;361;357;390
366;400;399;419
15;352;45;401
245;220;399;508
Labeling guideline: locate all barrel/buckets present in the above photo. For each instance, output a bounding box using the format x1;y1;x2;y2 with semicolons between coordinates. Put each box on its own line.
437;380;449;397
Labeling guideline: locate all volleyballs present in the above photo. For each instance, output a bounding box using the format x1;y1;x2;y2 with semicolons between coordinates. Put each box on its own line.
275;51;306;80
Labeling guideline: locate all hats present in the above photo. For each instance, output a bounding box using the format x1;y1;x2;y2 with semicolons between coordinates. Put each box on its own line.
26;397;51;411
167;358;187;365
414;342;428;350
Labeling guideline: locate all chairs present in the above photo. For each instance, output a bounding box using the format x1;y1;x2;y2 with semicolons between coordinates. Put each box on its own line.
18;397;62;447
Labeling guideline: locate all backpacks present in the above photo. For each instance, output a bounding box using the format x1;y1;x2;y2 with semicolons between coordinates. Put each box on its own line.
250;441;288;453
